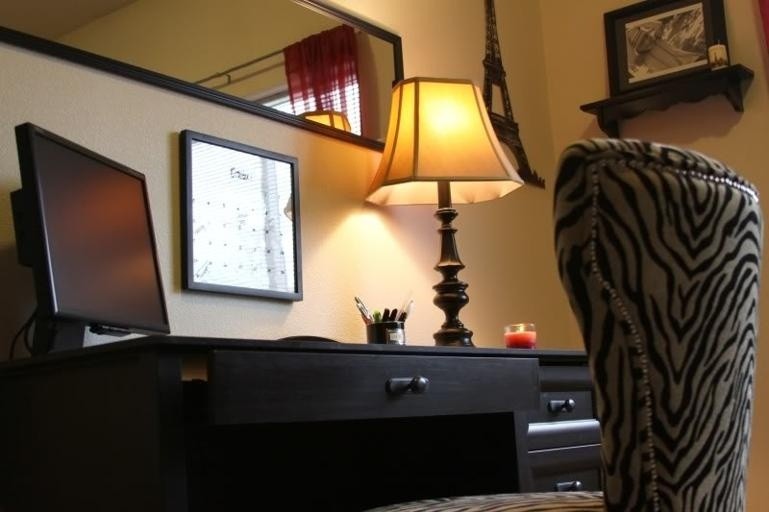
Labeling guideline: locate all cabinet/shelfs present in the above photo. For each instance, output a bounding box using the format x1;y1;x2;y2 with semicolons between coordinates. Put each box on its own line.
0;336;601;511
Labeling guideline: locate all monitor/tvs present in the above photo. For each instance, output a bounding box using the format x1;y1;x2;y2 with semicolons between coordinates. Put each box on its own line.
9;122;170;351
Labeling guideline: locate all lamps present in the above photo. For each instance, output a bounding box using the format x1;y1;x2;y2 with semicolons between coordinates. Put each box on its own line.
365;77;527;347
299;111;353;133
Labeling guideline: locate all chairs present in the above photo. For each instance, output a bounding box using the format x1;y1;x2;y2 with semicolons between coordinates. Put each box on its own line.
373;135;764;510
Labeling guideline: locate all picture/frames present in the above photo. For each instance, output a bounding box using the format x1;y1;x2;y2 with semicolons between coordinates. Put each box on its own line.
601;1;731;99
178;129;304;303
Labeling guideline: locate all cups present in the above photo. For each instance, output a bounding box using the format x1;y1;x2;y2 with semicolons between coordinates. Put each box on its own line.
365;319;406;345
502;322;538;350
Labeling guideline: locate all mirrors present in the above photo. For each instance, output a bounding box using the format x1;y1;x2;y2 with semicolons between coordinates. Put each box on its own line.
0;0;405;157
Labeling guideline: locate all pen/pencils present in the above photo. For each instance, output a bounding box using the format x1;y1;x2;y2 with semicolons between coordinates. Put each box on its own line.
354;296;413;324
